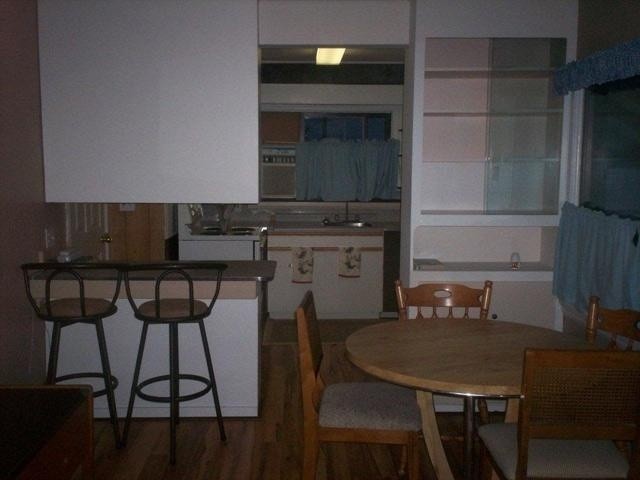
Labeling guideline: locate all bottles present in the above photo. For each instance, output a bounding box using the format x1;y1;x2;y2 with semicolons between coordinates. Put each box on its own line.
511;253;521;270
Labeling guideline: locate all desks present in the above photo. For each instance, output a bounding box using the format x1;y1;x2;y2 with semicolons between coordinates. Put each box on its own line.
346;316;601;479
22;383;94;465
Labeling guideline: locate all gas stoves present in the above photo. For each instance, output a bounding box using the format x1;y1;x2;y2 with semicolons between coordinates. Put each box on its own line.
190;225;259;236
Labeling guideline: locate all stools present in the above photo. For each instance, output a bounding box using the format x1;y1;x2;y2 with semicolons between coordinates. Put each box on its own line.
23;259;122;447
119;259;230;465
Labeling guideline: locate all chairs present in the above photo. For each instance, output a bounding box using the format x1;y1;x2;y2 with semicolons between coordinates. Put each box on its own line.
478;346;639;478
589;293;639;351
295;289;423;479
394;280;493;445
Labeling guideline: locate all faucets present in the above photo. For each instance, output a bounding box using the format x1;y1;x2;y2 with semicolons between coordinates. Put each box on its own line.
345;201;349;222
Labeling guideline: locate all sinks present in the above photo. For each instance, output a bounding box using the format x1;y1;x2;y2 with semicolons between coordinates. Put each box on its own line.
328;221;370;228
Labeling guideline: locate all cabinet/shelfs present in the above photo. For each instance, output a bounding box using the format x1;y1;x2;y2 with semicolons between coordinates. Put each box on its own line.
398;0;582;335
